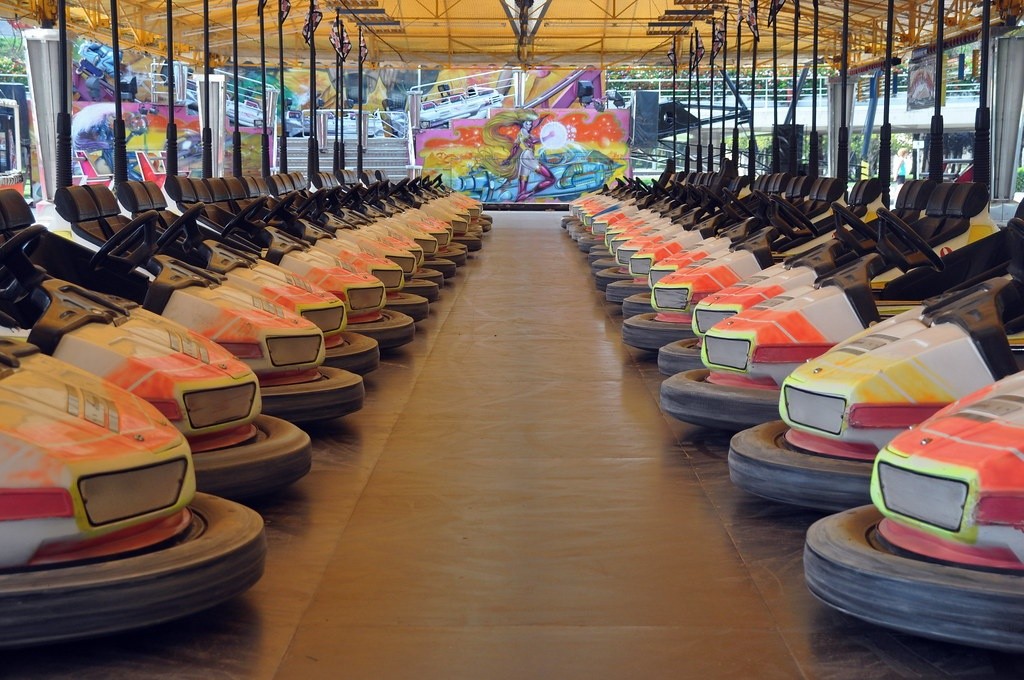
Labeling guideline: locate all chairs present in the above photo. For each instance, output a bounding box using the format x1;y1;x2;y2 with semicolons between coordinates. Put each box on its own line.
647;163;1024;294
438;84;451;97
245;87;254;102
0;150;399;326
318;99;324;108
285;98;293;109
382;99;393;111
347;99;354;109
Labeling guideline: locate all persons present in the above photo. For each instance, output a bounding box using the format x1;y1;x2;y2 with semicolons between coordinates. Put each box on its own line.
893;148;912;185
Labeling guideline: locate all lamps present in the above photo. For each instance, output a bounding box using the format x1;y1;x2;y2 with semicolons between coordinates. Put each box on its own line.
607;91;625;108
577;81;594;104
592;98;605;112
